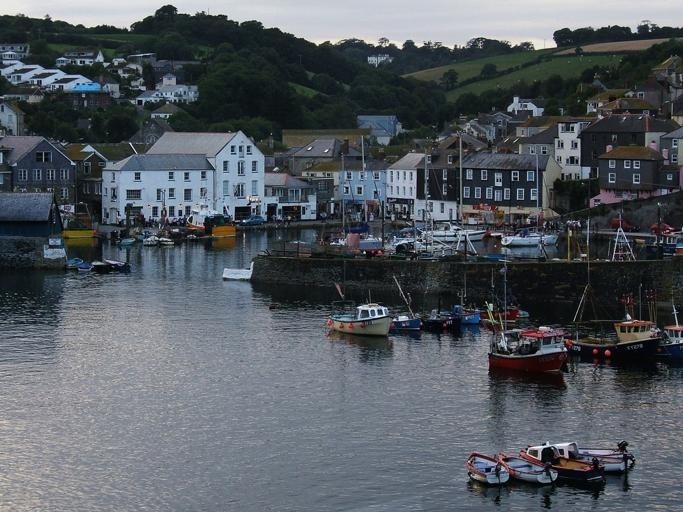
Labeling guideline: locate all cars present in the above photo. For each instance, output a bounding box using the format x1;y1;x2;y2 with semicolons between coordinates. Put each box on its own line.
240;214;263;226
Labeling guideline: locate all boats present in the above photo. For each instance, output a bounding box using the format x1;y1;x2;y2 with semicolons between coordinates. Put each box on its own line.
487;330;568;376
481;317;516;332
113;231;175;246
65;257;130;274
480;304;531;323
561;216;664;366
421;313;463;333
223;262;253;281
389;317;421;332
329;303;391;337
501;227;559;248
468;439;634;486
656;304;683;364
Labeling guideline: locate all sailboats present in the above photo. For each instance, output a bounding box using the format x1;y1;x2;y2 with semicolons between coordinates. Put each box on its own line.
290;134;488;261
436;234;480;326
